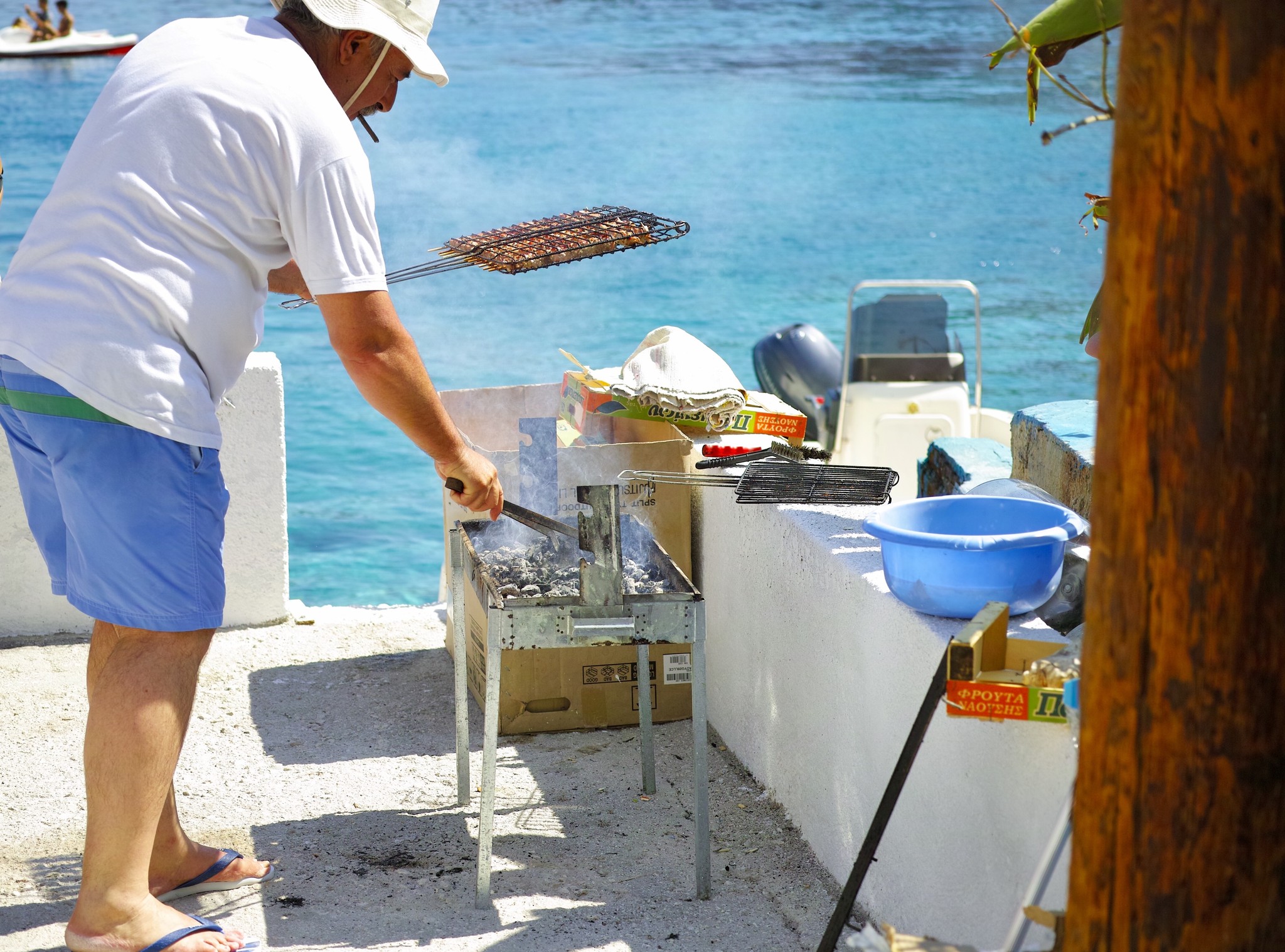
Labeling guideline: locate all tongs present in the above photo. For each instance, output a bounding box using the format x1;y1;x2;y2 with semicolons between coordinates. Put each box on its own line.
444;477;579;553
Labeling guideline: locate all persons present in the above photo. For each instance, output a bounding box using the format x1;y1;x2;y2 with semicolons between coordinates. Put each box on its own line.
0;0;508;952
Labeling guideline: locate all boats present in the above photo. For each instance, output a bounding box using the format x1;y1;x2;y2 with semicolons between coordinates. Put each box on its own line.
0;25;137;59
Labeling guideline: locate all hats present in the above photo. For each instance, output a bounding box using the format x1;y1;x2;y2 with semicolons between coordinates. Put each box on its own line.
266;0;450;89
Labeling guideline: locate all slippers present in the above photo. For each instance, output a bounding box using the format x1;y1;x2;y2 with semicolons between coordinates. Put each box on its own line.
141;913;260;952
156;848;274;903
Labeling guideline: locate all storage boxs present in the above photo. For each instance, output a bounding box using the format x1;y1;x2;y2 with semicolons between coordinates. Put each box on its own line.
946;601;1081;726
437;347;807;735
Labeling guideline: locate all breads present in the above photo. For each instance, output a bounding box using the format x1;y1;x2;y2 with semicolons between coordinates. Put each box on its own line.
1021;644;1081;688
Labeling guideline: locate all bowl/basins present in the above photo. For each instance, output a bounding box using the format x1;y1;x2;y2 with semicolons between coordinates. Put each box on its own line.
862;494;1085;619
